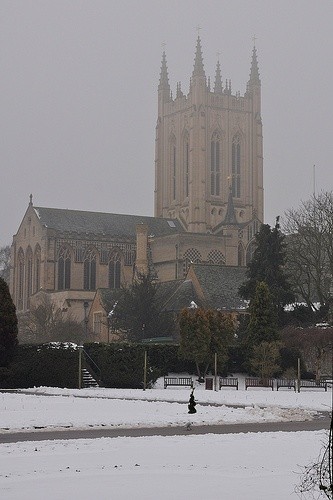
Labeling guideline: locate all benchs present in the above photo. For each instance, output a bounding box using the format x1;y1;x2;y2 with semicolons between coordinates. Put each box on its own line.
277;379;296;391
298;378;327;393
244;377;274;391
218;377;238;391
163;376;194;391
